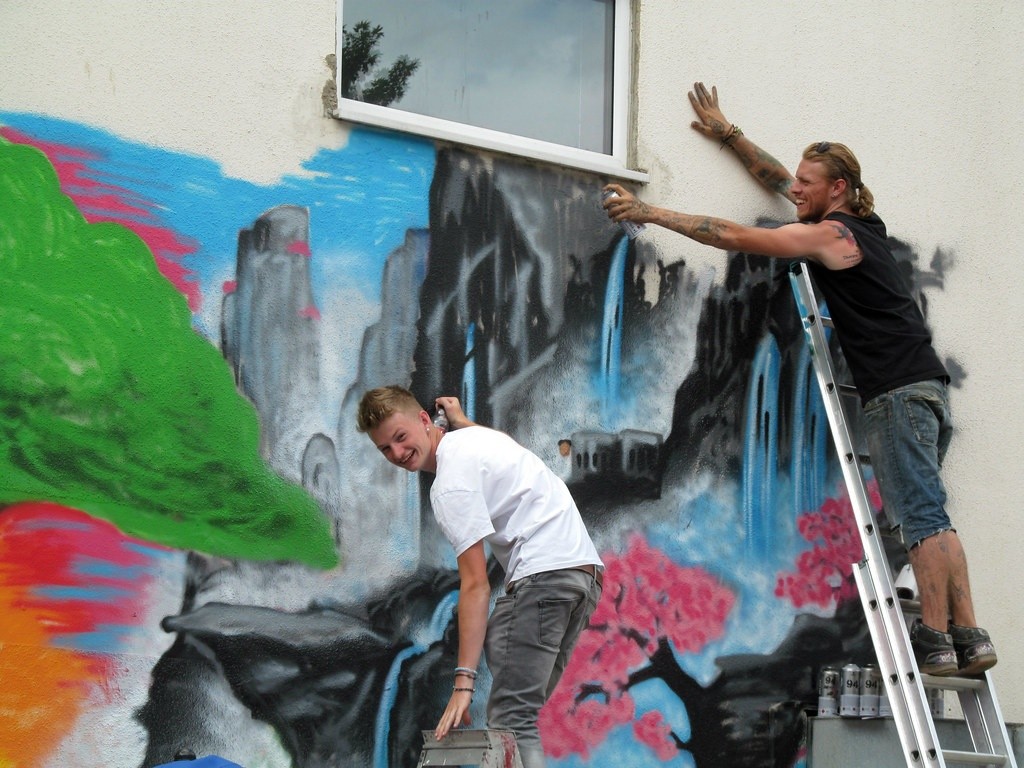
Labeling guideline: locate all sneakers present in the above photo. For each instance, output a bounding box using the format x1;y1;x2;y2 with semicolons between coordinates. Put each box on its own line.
947;622;997;677
909;617;958;677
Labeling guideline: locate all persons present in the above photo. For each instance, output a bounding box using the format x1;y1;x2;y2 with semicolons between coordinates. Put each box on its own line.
603;82;997;678
355;385;607;768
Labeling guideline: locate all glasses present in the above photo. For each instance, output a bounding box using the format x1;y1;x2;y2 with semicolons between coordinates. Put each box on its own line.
816;141;844;179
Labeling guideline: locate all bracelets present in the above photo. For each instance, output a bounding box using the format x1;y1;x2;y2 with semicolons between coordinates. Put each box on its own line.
454;667;478;675
452;685;475;691
454;673;476;680
721;124;741;146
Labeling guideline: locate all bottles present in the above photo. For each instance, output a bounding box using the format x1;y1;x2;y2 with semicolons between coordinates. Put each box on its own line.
600;185;649;240
818;661;943;717
430;407;449;434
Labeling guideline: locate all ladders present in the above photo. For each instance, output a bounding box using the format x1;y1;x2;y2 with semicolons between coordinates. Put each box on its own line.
787;259;1018;768
